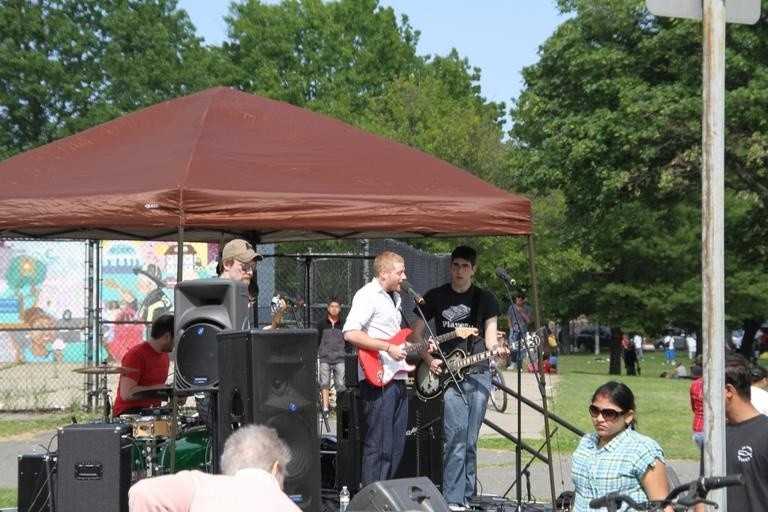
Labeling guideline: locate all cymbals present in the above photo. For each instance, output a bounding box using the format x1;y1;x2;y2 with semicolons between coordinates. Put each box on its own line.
132;386;174;399
73;367;138;375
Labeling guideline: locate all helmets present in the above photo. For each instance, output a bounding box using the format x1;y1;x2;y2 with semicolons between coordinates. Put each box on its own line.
133;264;166;289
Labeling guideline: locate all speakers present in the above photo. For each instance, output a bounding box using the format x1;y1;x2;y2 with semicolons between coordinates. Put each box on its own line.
56;422;132;512
393;388;444;495
346;475;450;512
173;279;251;397
216;327;322;512
336;388;363;495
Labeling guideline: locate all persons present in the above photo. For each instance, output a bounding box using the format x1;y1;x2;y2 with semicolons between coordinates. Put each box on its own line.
620;330;768;512
341;250;440;488
111;313;176;417
120;264;172;323
124;419;305;512
215;238;264;288
412;244;511;512
569;380;676;512
21;307;64;362
248;280;259;309
506;293;532;373
316;298;346;422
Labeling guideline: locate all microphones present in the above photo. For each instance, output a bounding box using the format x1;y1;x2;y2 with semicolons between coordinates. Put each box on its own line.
495;267;516;286
401;281;425;306
274;291;304;306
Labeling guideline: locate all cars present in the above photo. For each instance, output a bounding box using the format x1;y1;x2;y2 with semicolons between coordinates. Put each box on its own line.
554;322;768;354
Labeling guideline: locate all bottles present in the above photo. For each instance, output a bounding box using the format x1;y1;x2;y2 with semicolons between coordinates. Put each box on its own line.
339;486;351;512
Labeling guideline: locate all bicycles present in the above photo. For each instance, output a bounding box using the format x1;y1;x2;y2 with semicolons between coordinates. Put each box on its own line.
490;361;509;414
582;472;751;512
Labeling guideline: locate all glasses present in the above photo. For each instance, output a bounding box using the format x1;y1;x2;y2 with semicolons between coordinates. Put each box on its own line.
589;405;625;421
235;262;257;272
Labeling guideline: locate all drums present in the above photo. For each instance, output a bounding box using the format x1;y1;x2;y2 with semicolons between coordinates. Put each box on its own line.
132;416;180;437
159;426;214;475
120;413;141;424
131;437;168;470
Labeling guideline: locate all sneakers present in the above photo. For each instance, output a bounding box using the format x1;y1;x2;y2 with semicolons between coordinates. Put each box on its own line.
320;412;328;421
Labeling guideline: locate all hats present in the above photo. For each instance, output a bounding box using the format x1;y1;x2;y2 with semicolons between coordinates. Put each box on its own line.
222;239;263;263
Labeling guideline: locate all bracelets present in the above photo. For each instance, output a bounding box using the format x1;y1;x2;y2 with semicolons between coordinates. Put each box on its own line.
385;343;391;353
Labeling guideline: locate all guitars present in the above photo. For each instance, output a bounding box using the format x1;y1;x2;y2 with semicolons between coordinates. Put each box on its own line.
356;324;479;387
414;328;559;401
